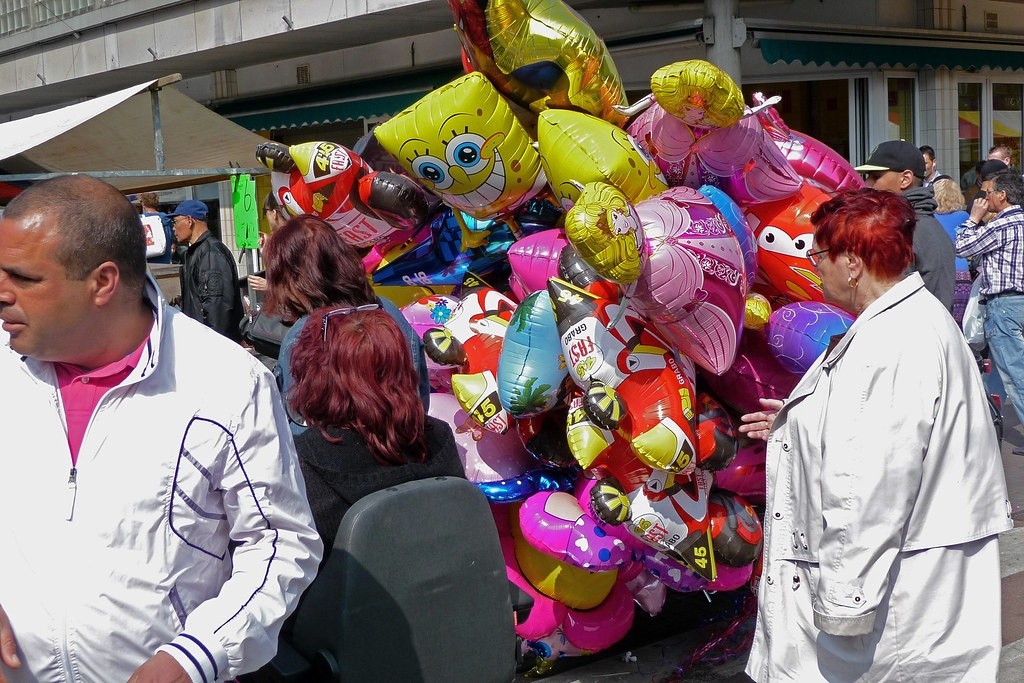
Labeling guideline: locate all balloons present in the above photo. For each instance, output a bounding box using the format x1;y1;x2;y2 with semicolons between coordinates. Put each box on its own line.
256;0;869;683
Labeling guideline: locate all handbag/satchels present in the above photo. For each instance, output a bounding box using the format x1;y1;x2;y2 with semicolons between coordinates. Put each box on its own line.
139;213;166;260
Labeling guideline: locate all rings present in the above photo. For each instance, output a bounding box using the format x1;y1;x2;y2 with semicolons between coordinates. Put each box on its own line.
766;422;768;428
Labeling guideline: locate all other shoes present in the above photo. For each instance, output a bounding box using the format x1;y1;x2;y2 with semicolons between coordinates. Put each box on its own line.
1012;445;1024;457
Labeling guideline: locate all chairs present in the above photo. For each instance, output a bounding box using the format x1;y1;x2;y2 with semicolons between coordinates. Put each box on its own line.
238;478;534;683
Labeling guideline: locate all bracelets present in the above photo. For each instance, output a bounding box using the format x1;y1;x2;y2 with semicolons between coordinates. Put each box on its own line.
966;219;978;225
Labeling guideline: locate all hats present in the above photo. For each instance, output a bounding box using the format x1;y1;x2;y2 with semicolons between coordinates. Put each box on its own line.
165;199;210;222
980;158;1007;181
851;138;927;179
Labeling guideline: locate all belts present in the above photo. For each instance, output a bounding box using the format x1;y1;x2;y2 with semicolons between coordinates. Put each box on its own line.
976;290;1024;305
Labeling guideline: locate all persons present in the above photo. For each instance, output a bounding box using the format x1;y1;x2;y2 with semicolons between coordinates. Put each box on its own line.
852;137;956;313
247;188;288;296
139;191;175;265
0;174;324;683
291;304;468;588
923;176;973;324
952;144;1024;460
169;237;182;264
736;192;1014;683
130;199;141;215
265;214;431;433
165;199;244;345
917;144;941;188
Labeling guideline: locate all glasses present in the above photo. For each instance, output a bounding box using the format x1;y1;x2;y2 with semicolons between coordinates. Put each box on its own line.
984;188;999;194
170;216;187;227
321;302;392;340
807;248;831;268
